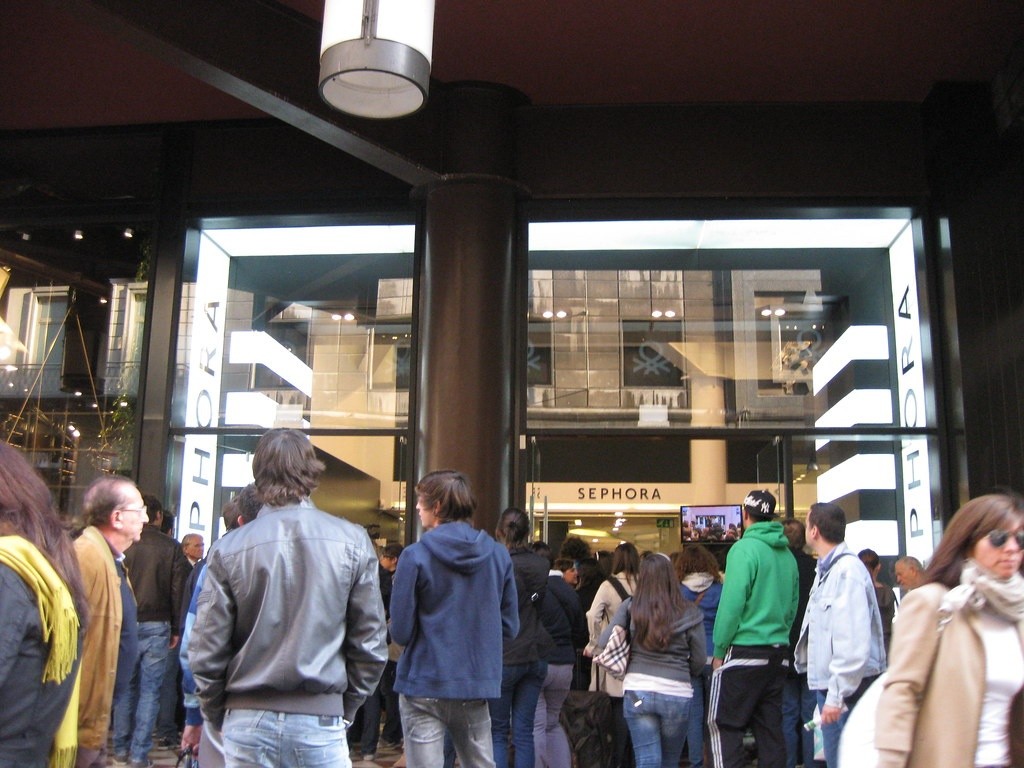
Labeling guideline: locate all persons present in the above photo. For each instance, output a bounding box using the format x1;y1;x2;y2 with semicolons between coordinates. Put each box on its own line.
0;425;926;768
875;493;1024;768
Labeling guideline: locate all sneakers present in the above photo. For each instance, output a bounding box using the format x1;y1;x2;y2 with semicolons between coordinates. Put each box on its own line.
112;754;128;765
348;733;404;761
131;758;155;768
158;734;181;750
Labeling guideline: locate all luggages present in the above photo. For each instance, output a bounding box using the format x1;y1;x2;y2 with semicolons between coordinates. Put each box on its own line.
559;648;620;768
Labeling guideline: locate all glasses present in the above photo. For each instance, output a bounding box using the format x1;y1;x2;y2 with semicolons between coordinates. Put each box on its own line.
987;528;1024;550
119;505;148;518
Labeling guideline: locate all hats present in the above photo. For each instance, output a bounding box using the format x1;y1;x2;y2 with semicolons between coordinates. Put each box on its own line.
743;489;780;519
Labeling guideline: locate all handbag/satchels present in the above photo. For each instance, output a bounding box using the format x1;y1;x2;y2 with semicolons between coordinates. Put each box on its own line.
592;596;633;682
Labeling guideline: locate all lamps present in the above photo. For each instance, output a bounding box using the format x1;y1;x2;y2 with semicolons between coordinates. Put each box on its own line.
316;0;437;119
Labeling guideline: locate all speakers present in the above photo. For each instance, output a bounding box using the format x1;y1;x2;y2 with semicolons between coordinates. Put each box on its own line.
919;80;1004;202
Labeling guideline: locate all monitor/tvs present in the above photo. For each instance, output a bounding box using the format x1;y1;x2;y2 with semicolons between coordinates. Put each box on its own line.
679;505;743;545
